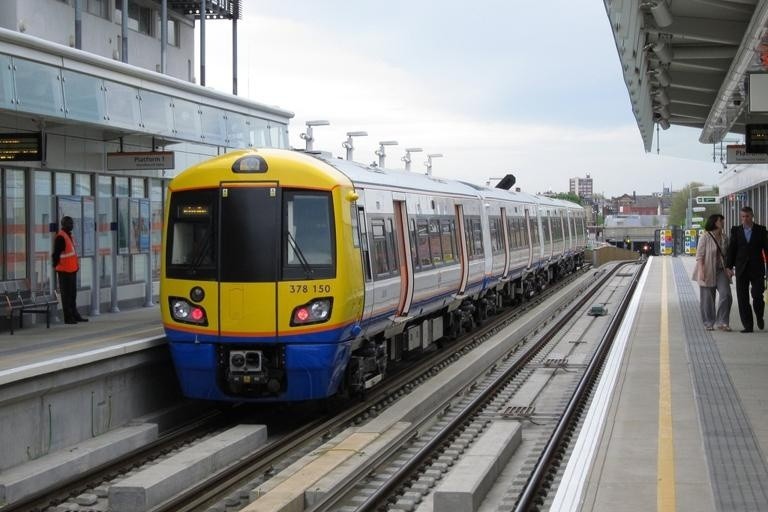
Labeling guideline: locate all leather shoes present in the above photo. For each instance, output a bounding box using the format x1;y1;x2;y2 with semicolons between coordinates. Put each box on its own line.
756;317;765;330
740;327;753;332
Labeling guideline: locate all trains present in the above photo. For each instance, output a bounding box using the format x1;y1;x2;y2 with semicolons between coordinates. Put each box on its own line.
159;147;587;403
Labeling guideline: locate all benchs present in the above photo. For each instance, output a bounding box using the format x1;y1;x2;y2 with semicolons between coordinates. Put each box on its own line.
1;278;60;335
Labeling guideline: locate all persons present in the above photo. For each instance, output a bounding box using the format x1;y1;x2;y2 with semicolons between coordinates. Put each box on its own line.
690;213;732;331
51;215;89;324
725;206;768;333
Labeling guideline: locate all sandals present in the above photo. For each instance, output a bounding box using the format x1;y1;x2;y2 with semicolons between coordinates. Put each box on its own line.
704;325;714;331
717;323;732;332
